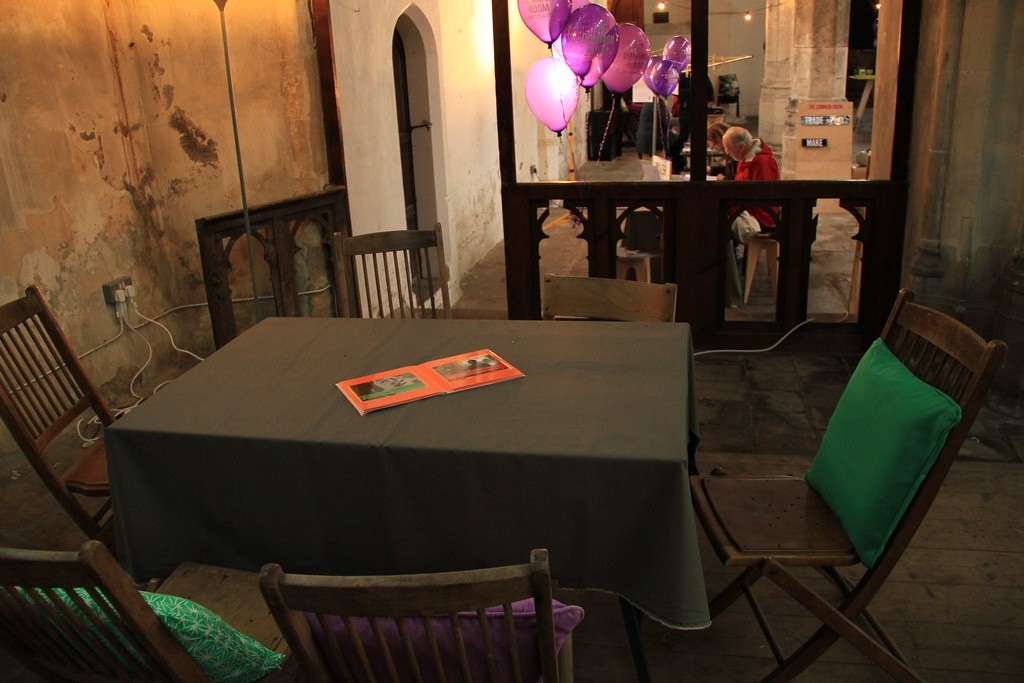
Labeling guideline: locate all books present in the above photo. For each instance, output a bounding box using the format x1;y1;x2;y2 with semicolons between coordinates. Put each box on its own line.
334;349;525;416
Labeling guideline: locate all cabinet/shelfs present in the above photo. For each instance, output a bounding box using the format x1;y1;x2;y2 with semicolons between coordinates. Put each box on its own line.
589;109;622;161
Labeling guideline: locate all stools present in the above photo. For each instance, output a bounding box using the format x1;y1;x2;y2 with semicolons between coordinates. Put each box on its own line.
743;232;778;306
616;252;662;284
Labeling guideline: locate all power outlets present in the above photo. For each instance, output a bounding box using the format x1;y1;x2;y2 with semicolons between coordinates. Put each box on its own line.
103;276;134;304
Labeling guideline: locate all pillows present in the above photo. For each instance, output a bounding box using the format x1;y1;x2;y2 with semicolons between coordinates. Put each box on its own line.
303;593;584;683
804;338;961;571
0;586;288;683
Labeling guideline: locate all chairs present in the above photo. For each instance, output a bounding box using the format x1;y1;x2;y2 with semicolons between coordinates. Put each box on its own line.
685;287;1006;683
0;559;306;683
542;273;678;324
0;285;117;564
260;547;576;683
333;223;453;319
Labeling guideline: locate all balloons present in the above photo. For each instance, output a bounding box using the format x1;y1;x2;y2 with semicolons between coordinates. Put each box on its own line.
662;35;691;73
518;1;590;60
598;23;651;98
525;59;580;137
562;3;619;93
643;55;663;98
651;60;680;100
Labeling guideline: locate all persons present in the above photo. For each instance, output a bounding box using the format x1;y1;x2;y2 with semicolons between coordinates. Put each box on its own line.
673;71;715;152
467;360;490;371
722;127;781;247
483;359;499;366
706;121;738;181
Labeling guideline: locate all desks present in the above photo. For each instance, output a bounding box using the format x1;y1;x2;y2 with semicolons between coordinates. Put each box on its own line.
680;144;730;158
849;75;877;132
104;315;698;683
